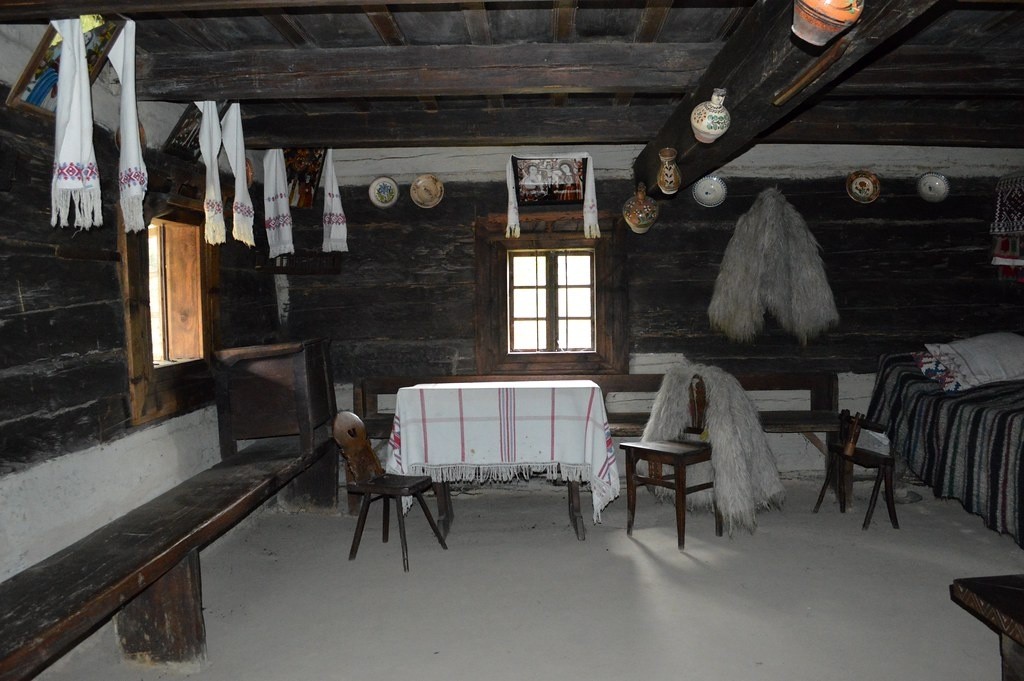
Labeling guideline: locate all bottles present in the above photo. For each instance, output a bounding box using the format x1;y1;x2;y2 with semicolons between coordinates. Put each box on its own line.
621;183;660;234
792;0;864;46
690;89;730;144
657;148;681;194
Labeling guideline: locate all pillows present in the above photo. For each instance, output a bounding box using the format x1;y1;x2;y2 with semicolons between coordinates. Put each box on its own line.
912;351;962;393
923;332;1024;385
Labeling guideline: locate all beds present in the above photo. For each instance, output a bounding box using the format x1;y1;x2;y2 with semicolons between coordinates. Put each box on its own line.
879;351;1023;552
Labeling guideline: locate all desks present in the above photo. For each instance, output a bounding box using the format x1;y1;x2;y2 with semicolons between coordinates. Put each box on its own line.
385;379;621;541
212;336;337;462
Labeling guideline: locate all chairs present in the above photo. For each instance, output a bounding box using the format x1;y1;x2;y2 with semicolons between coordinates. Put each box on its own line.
618;371;724;551
332;410;448;574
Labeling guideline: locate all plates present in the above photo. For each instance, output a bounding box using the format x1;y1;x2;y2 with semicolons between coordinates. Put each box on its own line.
410;173;445;208
114;121;148;157
917;172;949;202
245;156;254;188
368;176;400;208
846;171;881;203
691;176;727;207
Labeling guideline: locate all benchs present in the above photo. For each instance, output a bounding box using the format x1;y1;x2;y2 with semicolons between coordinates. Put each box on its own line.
347;372;853;500
0;417;347;681
812;414;900;530
